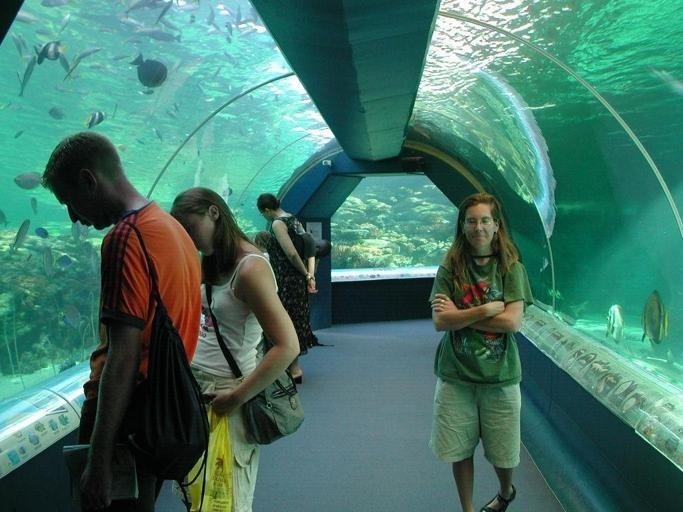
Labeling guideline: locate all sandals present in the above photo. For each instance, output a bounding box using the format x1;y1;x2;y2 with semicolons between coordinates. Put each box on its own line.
481;484;516;511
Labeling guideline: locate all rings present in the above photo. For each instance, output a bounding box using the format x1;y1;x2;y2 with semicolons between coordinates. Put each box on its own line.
440;299;445;303
440;304;444;307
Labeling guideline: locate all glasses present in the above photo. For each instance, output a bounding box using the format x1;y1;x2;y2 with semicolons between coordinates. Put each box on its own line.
462;218;494;226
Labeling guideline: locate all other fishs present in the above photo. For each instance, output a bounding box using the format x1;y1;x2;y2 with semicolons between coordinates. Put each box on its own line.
641;289;670;347
0;0;259;197
0;197;99;332
606;304;624;344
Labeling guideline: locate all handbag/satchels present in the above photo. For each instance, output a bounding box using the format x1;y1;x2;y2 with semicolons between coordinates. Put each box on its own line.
243;367;306;446
291;231;316;260
132;306;210;482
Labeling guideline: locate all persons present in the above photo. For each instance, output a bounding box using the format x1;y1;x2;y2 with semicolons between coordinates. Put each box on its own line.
39;129;204;512
170;184;301;512
252;230;272;262
427;191;534;512
255;191;319;385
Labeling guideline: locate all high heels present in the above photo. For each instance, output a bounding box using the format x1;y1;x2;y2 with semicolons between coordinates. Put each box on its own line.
293;376;303;384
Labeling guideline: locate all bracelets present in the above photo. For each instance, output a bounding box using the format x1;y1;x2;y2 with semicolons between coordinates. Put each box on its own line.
308;277;314;280
304;272;308;277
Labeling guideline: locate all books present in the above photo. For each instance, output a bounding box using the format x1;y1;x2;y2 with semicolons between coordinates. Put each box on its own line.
58;436;140;501
